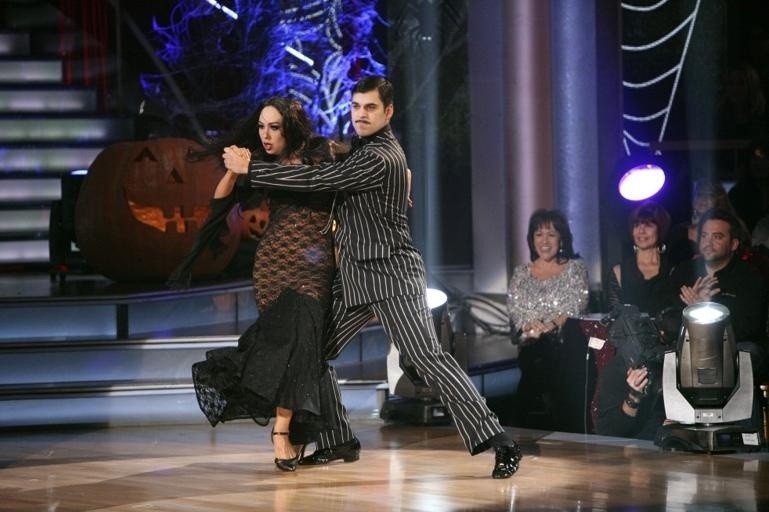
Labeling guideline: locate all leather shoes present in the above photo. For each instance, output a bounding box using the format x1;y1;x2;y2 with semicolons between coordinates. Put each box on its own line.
493;441;522;478
270;427;306;471
299;436;361;465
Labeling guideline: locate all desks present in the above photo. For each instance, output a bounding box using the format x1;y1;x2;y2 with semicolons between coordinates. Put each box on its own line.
567;312;650;426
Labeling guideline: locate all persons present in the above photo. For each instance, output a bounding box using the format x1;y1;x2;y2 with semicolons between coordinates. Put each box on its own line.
598;178;769;443
192;96;413;472
506;208;597;437
220;75;522;479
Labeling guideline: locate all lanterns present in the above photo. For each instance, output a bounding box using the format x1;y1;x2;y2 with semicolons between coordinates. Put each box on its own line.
73;137;241;283
240;199;271;243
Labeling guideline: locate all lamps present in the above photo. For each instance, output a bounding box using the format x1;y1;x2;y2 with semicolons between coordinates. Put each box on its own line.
50;169;89;284
614;140;769;202
425;287;448;343
662;302;764;454
